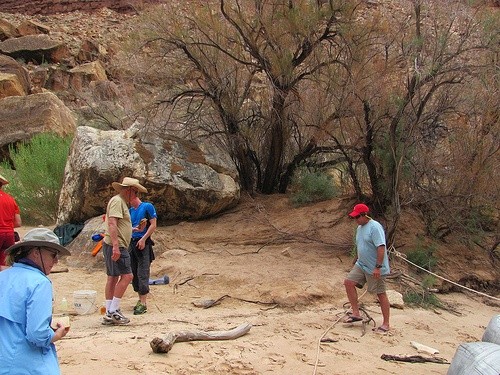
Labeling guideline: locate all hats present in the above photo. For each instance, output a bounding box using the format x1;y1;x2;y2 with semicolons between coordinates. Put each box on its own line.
349;203;369;217
5;228;71;256
112;177;148;193
0;175;9;184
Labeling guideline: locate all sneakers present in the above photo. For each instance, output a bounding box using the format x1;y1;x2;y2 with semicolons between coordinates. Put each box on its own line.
103;319;129;326
106;309;130;323
134;303;147;315
134;300;141;309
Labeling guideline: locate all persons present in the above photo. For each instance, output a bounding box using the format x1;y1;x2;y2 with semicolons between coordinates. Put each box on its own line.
128;197;157;315
0;174;20;267
102;177;148;325
0;228;72;375
343;204;391;332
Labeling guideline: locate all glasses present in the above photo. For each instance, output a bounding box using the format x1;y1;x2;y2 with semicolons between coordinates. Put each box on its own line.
355;213;365;219
129;187;138;195
36;247;57;258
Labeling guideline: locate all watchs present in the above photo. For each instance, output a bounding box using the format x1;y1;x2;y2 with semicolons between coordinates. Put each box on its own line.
376;264;382;268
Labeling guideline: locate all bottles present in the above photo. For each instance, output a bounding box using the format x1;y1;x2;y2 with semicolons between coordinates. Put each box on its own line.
61;298;68;312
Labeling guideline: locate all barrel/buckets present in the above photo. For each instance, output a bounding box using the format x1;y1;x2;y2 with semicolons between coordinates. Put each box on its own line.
72;290;98;315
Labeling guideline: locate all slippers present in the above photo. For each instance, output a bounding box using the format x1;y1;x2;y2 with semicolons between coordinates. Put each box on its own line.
344;316;362;322
372;326;389;332
